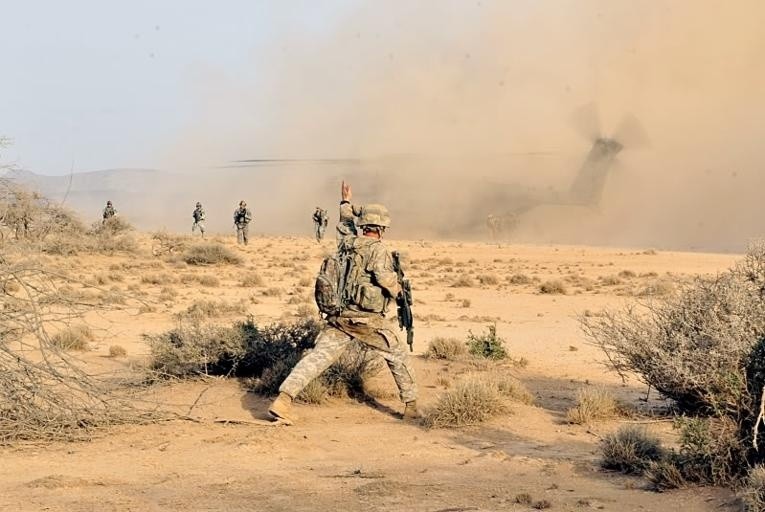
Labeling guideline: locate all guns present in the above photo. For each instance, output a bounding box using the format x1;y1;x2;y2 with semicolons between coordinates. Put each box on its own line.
314;214;321;226
390;251;413;352
234;209;246;223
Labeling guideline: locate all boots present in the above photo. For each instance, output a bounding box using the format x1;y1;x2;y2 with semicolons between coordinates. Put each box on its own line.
269;391;298;426
402;401;429;420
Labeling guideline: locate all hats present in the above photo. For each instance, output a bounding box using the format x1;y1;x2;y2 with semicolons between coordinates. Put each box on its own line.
240;200;246;207
107;201;112;205
355;202;391;228
196;202;201;206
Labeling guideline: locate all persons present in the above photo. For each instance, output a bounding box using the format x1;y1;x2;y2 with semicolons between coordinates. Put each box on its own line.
266;178;435;428
103;200;118;225
312;206;329;244
191;201;208;241
233;199;253;246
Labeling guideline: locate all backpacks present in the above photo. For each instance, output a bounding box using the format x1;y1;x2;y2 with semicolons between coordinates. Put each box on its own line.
315;234;382;315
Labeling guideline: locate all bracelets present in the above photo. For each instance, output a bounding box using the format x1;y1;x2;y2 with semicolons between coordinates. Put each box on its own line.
340;200;351;205
